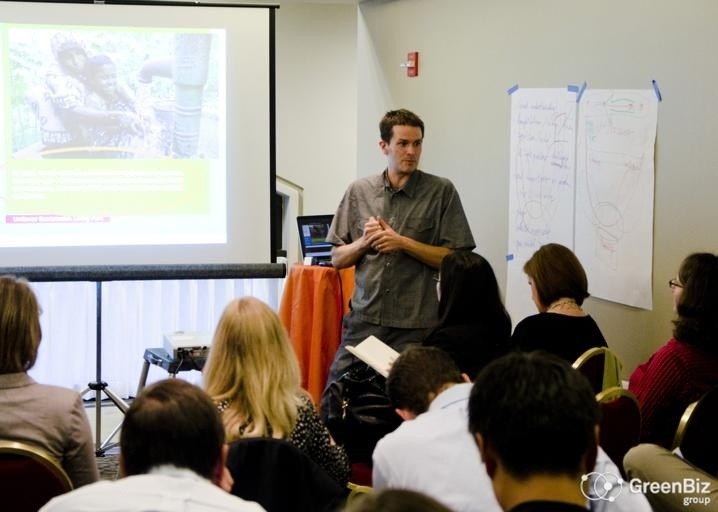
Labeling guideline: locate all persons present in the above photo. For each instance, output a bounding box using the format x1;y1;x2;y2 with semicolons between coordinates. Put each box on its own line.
325;110;476;463
78;53;158;147
0;243;718;511
34;36;154;147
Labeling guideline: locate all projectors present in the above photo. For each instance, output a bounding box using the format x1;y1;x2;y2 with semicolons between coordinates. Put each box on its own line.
163;331;215;361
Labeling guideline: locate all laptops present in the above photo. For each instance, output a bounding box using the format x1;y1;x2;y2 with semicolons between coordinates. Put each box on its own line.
295;214;335;266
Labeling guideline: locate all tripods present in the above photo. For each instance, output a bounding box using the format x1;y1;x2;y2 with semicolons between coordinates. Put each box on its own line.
79;282;130;456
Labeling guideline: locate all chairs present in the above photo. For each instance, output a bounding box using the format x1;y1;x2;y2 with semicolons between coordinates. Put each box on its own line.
595;387;644;479
568;347;622;397
671;393;718;478
0;439;77;512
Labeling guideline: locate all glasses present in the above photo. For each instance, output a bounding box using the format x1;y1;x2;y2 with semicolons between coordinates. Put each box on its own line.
669;279;684;288
